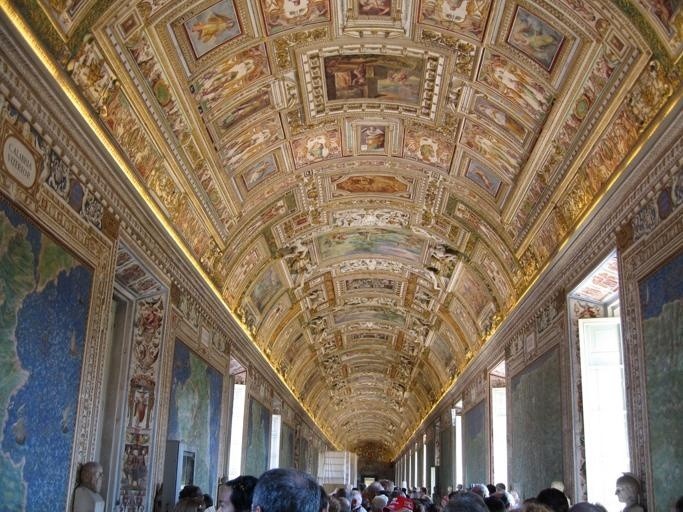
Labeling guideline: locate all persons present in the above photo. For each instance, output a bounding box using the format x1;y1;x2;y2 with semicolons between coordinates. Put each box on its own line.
615;473;644;512
72;463;105;512
